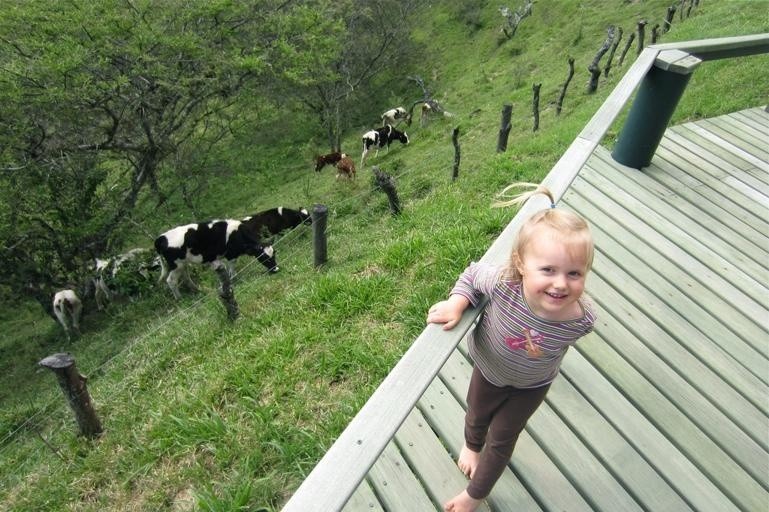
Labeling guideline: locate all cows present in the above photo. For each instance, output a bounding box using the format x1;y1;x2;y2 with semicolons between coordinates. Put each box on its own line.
314;151;351;173
154;218;280;304
359;124;410;169
335;153;357;184
53;288;84;344
380;106;407;126
421;98;439;123
86;246;165;312
242;206;312;239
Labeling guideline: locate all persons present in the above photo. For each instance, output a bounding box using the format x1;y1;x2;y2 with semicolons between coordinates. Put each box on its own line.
424;180;597;512
